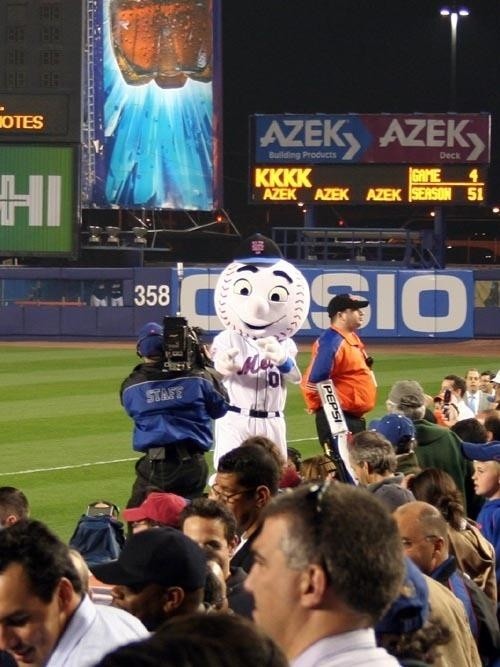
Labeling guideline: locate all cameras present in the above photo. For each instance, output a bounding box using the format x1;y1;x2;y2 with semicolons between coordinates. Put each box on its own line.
441;390;451;420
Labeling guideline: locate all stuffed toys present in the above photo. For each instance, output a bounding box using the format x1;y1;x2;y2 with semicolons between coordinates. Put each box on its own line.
210;232;311;482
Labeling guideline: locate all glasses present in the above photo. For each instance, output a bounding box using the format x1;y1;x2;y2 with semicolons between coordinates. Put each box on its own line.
211;484;246;503
401;534;437;547
305;480;331;583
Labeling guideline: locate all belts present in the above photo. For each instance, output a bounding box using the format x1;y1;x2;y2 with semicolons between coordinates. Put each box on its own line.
228;405;280;418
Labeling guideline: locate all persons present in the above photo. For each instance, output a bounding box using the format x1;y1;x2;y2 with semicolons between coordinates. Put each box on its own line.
1;369;499;665
91;281;109;307
117;323;232;539
110;280;124;307
28;279;48;300
301;293;378;485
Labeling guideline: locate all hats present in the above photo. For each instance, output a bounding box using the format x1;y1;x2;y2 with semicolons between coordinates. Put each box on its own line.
137;323;164;356
327;294;369;316
88;527;208;590
433;393;457;404
123;492;187;526
369;413;414;446
490;371;500;384
461;441;500;461
389;381;425;407
235;233;282;264
